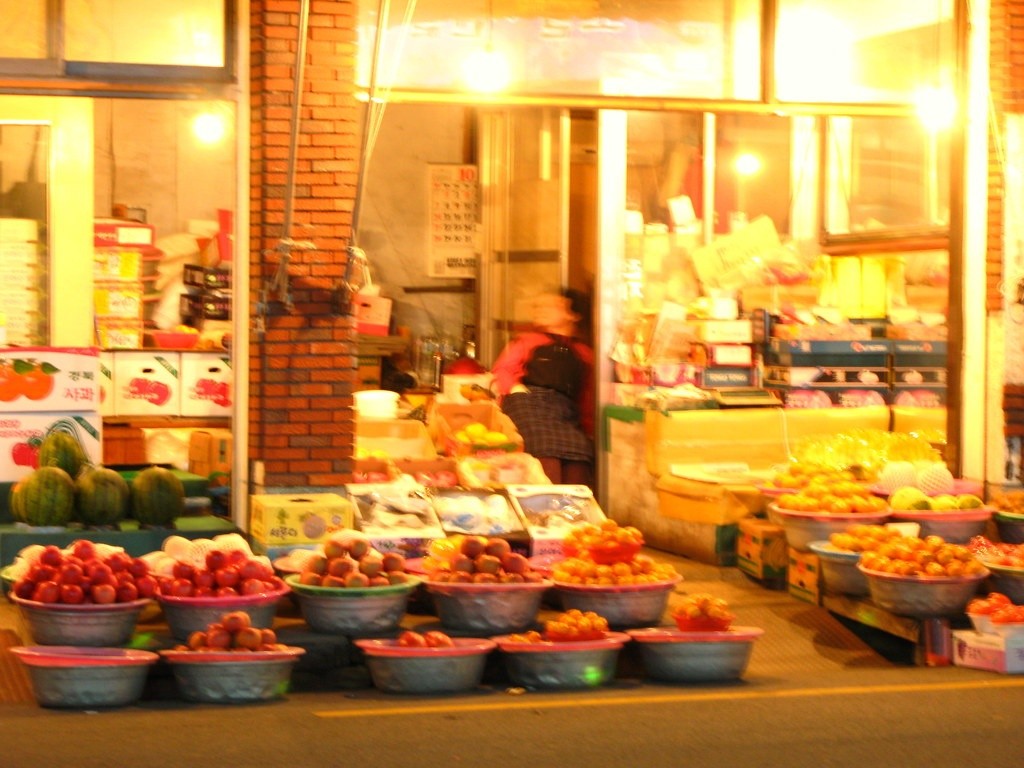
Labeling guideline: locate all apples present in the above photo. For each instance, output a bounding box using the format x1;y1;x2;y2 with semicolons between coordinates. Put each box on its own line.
434;536;541;582
15;540;157;605
159;549;281;597
173;611;278;653
398;628;453;648
299;538;407;589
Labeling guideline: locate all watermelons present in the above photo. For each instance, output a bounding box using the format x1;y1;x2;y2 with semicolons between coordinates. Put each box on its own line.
11;428;185;526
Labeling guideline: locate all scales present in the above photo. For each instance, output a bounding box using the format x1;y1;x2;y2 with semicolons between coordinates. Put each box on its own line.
701;384;783;407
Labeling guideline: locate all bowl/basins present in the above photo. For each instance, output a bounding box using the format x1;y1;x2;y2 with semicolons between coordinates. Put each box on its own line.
893;509;995;545
495;632;633;689
147;329;201;351
870;478;985;501
7;591;154;646
423;579;555;635
155;584;292;641
555;573;685;625
766;501;894;552
993;512;1024;544
158;646;306;701
0;566;19;586
983;563;1024;607
285;574;422;634
8;646;158;710
807;539;870;597
353;636;499;694
627;626;765;684
856;560;992;620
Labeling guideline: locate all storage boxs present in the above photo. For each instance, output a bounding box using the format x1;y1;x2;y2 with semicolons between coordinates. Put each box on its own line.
2;411;103;482
788;545;826;608
96;317;143;350
352;398;606;555
890;339;948;367
738;518;787;585
95;248;142;284
249;492;354;546
357;296;393;335
93;216;155;247
767;339;888;368
182;354;233;414
98;352;113;415
0;347;100;413
250;537;315;559
96;281;144;320
115;352;182;416
690;317;753;388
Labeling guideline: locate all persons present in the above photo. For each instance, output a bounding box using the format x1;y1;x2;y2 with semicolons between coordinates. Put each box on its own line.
489;286;597;495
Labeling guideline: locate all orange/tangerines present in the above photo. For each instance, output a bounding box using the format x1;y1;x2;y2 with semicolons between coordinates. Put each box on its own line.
551;519;676;585
772;460;982;575
